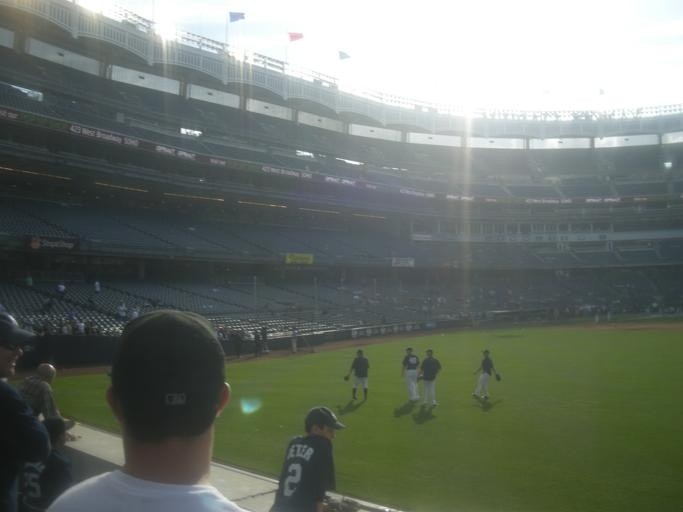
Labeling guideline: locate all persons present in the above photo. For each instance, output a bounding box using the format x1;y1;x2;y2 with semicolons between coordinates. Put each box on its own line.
418;348;443;406
469;349;502;400
17;416;72;512
344;350;371;400
269;404;346;511
39;310;251;511
1;266;683;358
400;346;421;401
1;308;53;512
18;364;79;434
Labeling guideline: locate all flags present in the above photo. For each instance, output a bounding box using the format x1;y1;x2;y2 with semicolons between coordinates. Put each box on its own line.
338;51;351;60
229;11;244;22
289;31;302;41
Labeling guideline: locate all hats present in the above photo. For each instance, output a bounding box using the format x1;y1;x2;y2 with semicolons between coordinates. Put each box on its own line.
43;419;74;436
0;313;37;345
112;310;225;406
306;407;345;429
